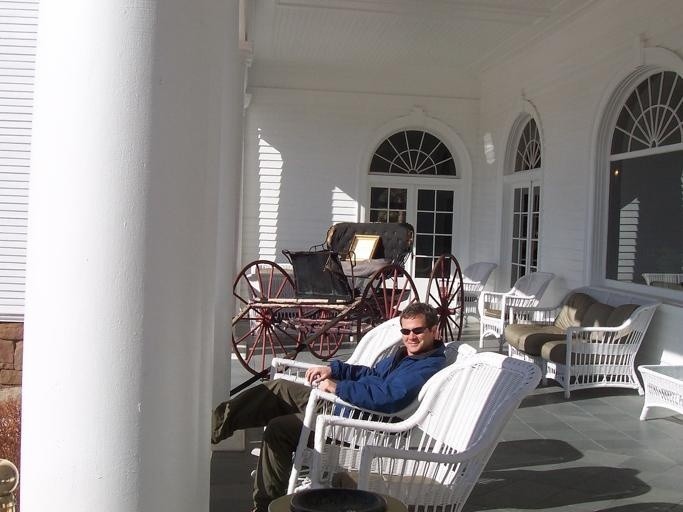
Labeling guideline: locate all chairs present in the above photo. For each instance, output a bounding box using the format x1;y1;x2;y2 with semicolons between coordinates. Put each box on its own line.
271;316;442;406
438;261;498;330
315;355;543;512
478;272;552;349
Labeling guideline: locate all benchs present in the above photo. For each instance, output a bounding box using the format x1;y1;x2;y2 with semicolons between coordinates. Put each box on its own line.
309;223;414;279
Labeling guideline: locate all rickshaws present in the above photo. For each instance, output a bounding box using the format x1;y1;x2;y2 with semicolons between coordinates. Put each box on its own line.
230;223;464;397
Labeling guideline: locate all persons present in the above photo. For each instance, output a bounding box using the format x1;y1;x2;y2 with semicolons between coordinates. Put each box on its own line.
210;302;445;511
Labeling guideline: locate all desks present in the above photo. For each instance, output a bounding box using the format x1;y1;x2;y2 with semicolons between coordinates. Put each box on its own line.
268;486;408;511
638;360;683;427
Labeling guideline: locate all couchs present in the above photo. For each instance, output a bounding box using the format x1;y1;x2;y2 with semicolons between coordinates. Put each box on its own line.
506;285;662;398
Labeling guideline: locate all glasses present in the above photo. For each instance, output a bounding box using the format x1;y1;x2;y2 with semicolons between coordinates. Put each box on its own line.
401;327;427;334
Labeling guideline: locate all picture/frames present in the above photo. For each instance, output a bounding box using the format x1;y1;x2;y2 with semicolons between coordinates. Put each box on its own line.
345;235;380;262
